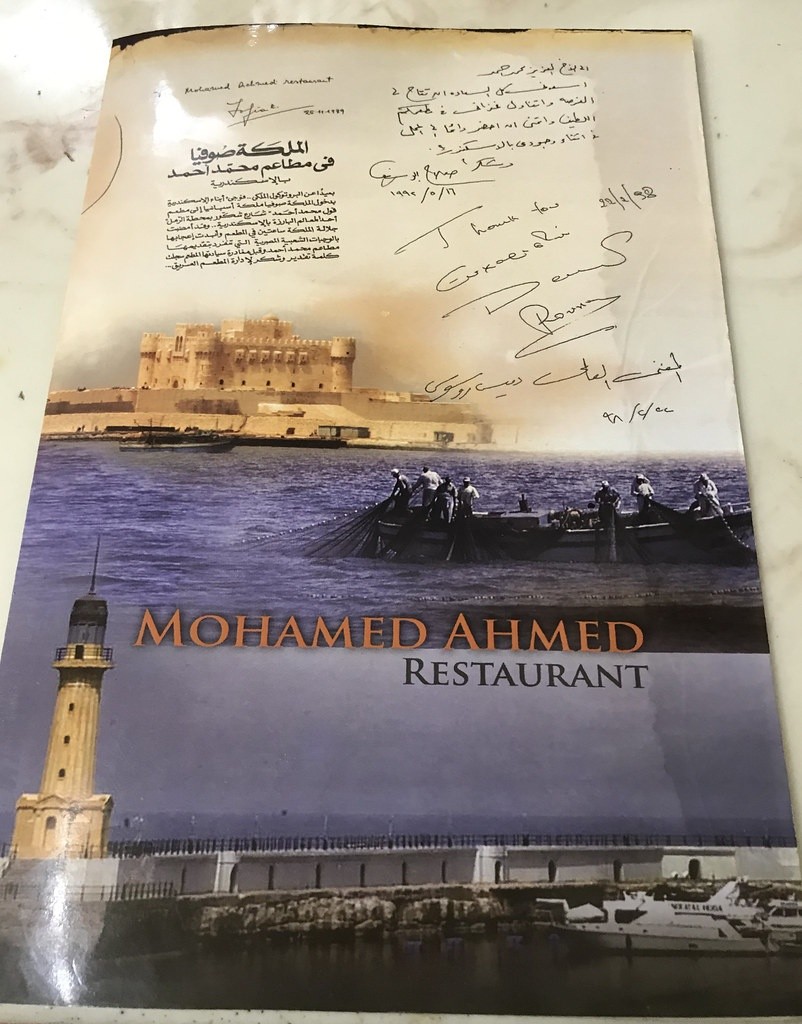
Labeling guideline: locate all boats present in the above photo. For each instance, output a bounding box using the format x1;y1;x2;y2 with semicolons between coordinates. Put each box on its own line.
119;417;240;453
541;927;802;957
377;494;757;563
534;876;802;942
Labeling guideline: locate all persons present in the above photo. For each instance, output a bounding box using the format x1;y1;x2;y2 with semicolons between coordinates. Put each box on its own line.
631;473;655;520
389;466;480;524
594;480;623;531
693;472;724;518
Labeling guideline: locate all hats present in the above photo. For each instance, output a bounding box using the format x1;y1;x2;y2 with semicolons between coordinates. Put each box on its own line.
700;473;710;481
463;477;470;482
